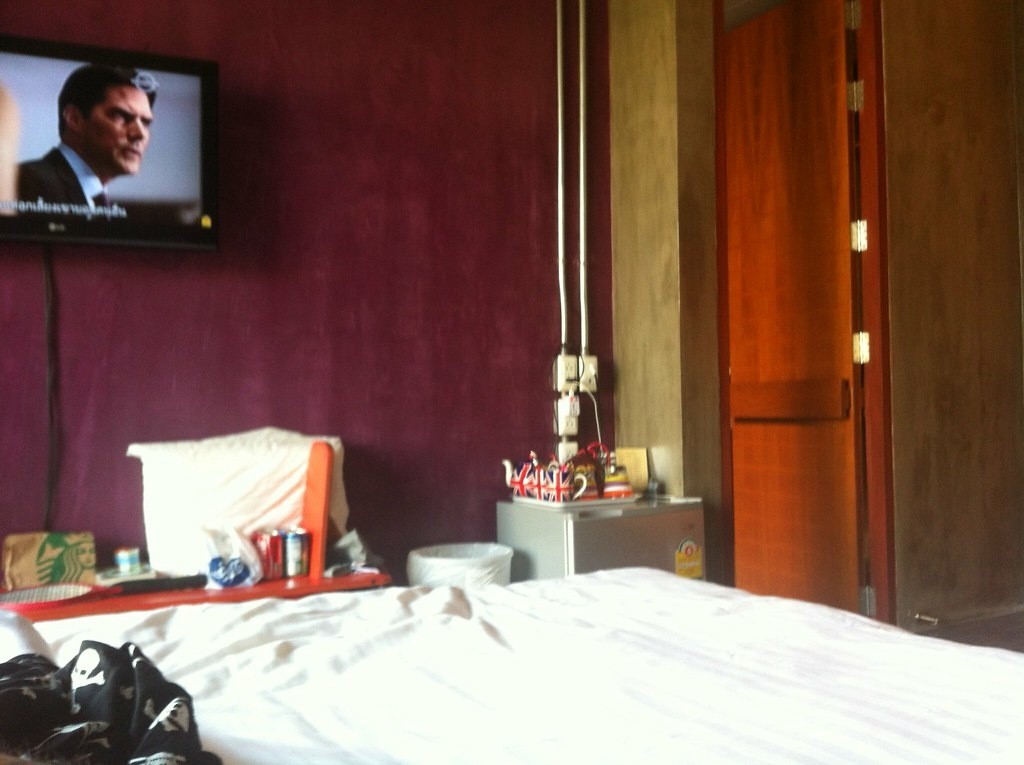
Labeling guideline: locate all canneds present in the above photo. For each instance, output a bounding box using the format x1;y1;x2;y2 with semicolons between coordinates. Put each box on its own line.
251;527;309;579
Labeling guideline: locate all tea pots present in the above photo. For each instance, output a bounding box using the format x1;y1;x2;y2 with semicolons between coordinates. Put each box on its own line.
501;451;588;502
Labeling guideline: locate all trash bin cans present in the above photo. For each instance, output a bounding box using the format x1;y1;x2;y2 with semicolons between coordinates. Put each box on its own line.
407;542;514;588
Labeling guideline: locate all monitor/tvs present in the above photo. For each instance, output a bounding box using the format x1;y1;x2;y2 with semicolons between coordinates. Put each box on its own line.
0;33;221;252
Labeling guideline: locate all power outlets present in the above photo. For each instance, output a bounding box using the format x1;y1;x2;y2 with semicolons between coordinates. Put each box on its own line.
558;442;578;465
557;399;578;436
557;355;578;393
579;355;598;393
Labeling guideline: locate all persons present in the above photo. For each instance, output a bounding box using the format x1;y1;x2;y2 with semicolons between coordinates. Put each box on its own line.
14;62;158;212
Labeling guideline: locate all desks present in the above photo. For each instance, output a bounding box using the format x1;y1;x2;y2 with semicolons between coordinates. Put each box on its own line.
17;572;393;623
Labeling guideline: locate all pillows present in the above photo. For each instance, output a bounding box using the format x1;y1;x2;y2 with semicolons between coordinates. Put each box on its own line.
126;426;350;573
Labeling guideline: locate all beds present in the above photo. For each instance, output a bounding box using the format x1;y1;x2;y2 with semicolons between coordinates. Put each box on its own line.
0;566;1024;765
